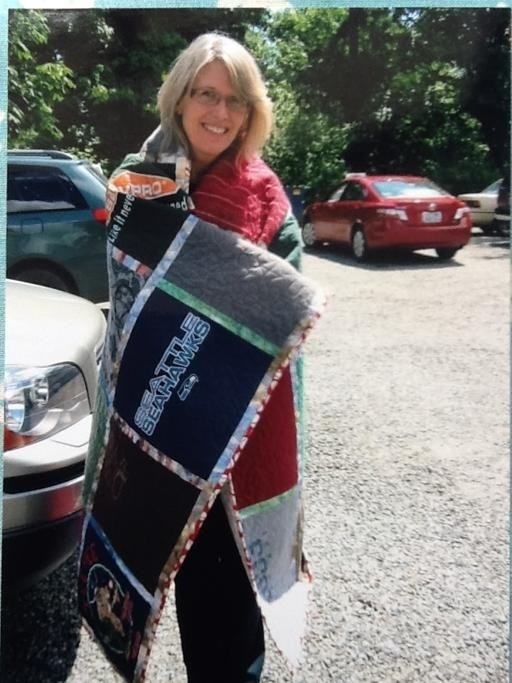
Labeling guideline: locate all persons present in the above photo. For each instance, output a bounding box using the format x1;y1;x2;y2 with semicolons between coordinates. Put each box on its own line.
80;31;331;681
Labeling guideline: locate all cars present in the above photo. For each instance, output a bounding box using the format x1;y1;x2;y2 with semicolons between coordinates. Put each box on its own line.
462;175;504;239
297;170;481;264
4;274;113;607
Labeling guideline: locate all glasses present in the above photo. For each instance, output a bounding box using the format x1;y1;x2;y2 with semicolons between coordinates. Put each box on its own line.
183;84;252;113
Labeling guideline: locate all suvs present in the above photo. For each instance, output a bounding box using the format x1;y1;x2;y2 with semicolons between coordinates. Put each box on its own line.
5;143;125;309
